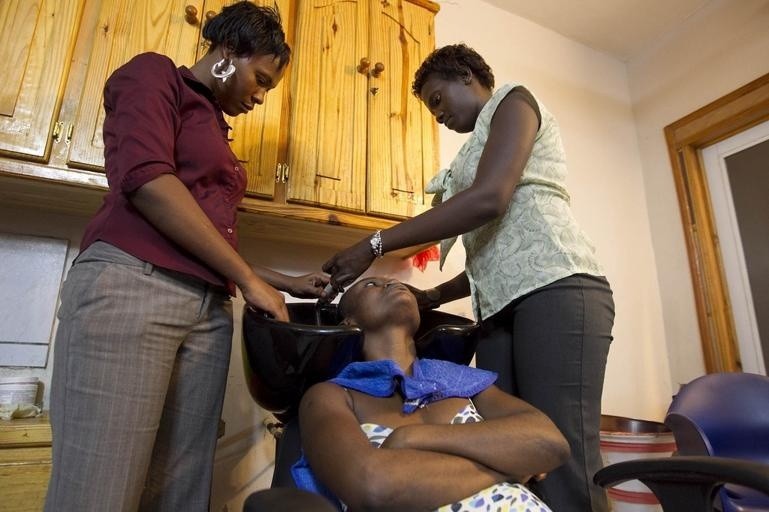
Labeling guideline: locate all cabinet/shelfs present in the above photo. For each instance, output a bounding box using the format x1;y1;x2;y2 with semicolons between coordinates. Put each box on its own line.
64;0;288;238
281;2;441;261
0;0;83;216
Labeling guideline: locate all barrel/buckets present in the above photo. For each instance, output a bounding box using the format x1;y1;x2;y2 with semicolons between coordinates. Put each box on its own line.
599;415;679;512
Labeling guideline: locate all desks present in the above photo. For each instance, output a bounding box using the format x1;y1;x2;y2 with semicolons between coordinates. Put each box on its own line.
0;411;52;511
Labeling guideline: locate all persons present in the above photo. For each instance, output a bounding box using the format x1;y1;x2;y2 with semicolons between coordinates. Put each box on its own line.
298;273;572;511
322;40;615;512
42;0;346;510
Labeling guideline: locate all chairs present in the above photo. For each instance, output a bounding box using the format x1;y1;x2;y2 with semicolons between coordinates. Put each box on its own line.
592;371;769;512
243;438;341;512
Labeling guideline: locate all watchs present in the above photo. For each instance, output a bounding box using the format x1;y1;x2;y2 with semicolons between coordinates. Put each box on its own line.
370;228;383;260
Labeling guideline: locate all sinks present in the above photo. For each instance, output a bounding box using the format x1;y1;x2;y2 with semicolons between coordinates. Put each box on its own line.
240;300;487;430
